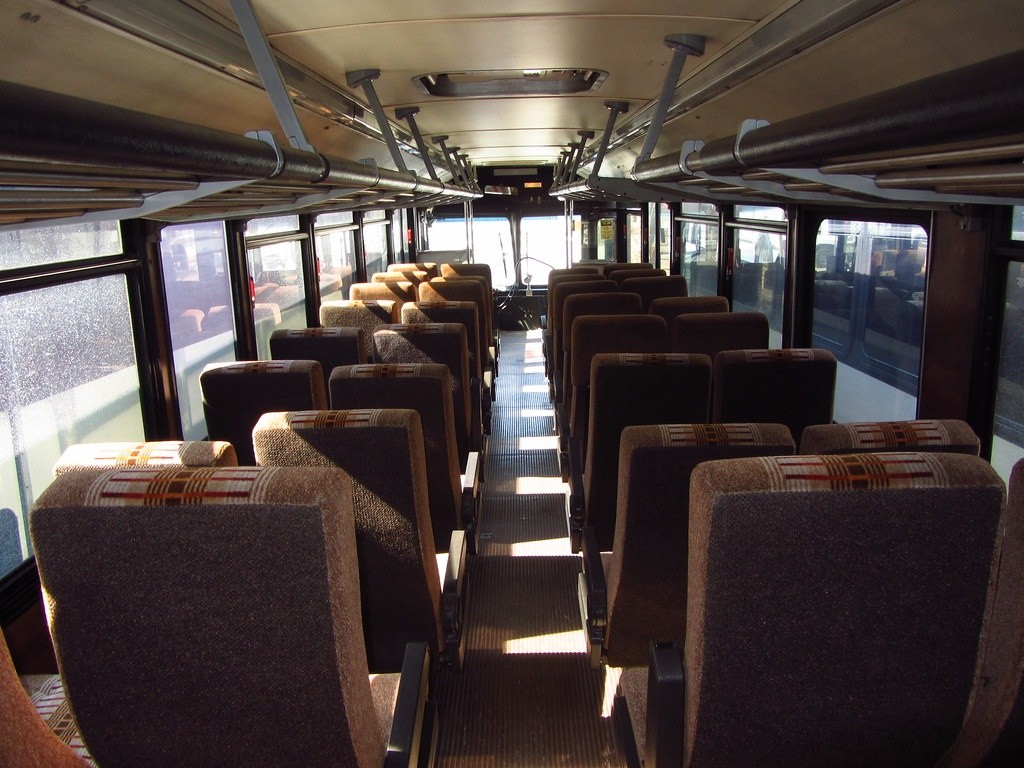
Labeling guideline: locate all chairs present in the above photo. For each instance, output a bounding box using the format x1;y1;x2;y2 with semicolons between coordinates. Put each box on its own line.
0;261;1024;768
